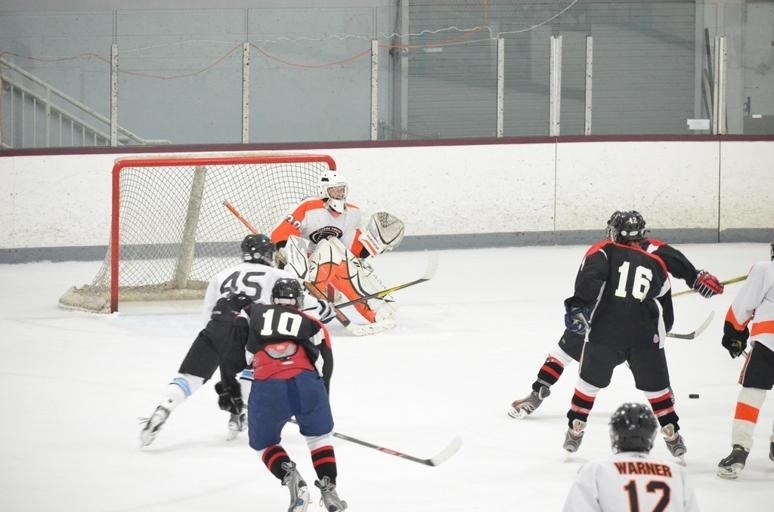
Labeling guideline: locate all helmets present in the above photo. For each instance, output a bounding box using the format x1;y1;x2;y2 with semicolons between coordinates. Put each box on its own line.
610;403;656;453
318;170;348;213
242;235;274;266
606;211;648;244
272;279;305;310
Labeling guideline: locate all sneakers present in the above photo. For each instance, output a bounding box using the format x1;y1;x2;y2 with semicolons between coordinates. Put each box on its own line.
321;488;347;510
667;438;687;457
145;406;169;431
229;414;242;428
718;450;749;466
286;471;306;512
511;393;542;416
564;432;582;452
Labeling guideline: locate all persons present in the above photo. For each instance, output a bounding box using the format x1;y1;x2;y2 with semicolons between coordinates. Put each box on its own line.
560;210;687;455
139;233;290;446
270;168;396;327
511;208;723;416
562;403;702;511
717;238;773;472
217;277;348;512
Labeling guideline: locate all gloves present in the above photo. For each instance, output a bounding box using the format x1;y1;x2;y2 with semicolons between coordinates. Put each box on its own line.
693;270;723;297
565;308;589;336
722;321;749;358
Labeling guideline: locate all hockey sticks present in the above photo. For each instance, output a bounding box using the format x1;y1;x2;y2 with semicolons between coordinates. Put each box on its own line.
299;249;439;314
663;306;721;341
222;199;396;336
240;402;473;468
